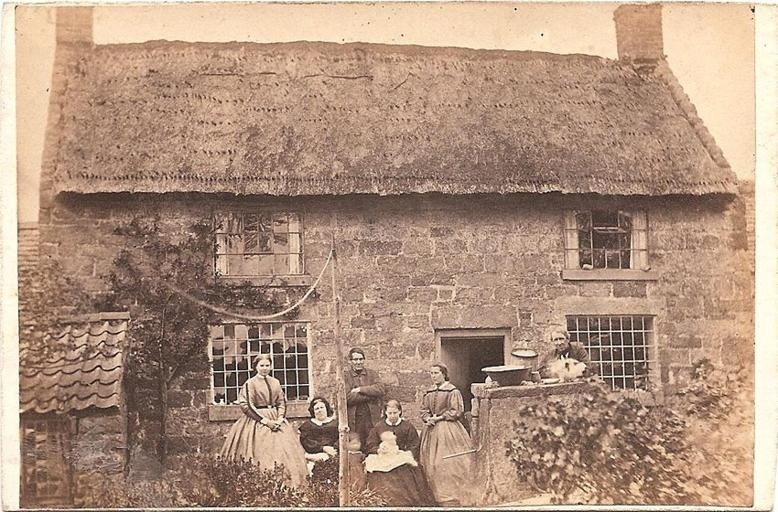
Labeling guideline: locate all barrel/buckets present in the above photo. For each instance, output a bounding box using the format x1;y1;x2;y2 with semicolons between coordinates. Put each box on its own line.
511;347;539;372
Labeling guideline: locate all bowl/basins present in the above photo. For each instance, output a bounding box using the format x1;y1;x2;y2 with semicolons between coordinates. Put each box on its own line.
482;364;532;387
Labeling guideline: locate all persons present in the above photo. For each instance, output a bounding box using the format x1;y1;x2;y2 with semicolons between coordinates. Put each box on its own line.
298;397;339;477
536;328;592;383
217;353;309;498
364;399;438;507
346;432;368;494
419;364;475;507
345;348;388;457
